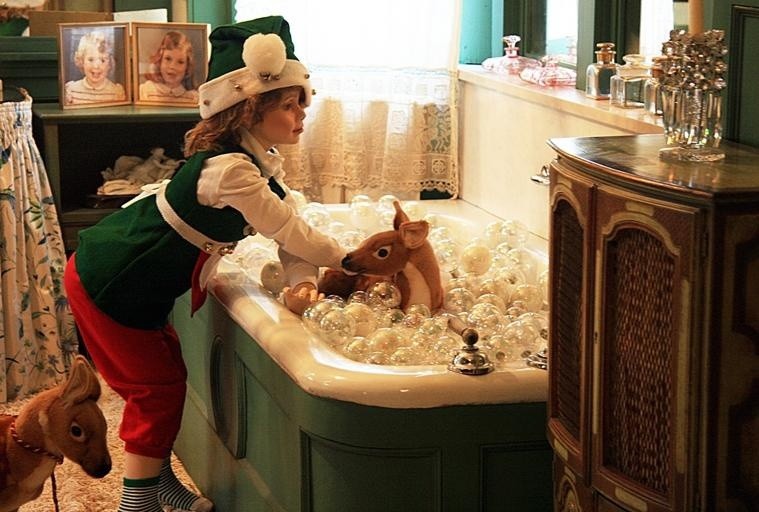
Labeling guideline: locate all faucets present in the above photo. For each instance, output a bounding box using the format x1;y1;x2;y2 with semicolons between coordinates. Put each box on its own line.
446;315;494;374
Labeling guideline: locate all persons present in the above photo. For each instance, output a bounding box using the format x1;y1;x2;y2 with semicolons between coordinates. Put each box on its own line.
59;14;352;512
63;31;130;104
137;31;203;106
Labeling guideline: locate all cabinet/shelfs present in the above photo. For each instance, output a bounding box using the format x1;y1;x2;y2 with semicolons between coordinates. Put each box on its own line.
28;101;205;252
542;137;758;512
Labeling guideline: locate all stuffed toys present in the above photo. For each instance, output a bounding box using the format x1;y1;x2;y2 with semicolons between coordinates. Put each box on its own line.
0;354;112;512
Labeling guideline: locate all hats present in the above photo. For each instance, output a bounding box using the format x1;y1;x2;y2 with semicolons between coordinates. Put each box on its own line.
199;16;315;121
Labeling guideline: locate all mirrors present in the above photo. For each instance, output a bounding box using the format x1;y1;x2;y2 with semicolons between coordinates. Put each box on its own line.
618;0;690;79
519;0;580;71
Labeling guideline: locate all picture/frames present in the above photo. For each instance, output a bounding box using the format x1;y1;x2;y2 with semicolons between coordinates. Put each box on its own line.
129;20;211;108
55;21;133;110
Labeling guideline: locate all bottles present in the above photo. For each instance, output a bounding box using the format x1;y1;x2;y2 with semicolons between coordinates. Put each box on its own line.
481;36;577;87
582;40;663;116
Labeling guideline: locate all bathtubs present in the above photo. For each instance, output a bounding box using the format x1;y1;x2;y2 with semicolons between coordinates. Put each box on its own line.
209;199;546;409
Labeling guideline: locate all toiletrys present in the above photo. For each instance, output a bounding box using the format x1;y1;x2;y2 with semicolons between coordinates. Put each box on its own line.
585;44;619;101
499;36;544;76
